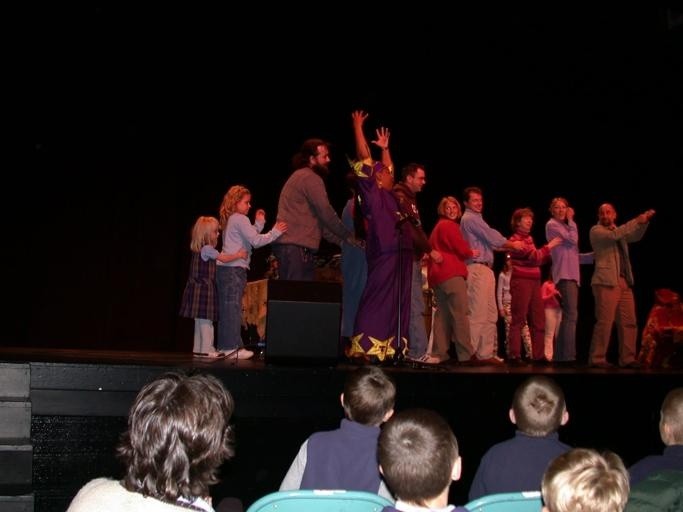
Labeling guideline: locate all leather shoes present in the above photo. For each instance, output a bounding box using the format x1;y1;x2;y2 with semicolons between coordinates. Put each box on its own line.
590;364;613;369
480;358;501;364
625;361;640;368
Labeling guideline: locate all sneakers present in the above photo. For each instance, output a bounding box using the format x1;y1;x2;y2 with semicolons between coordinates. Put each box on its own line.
193;347;253;360
410;354;440;363
459;354;480;367
508;357;527;367
554;360;582;367
494;356;504;362
532;359;550;367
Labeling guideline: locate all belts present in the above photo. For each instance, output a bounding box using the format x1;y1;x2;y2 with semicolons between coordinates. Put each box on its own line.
473;261;488;266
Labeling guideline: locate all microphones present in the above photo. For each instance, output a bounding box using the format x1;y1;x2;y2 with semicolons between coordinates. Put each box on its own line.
405;212;421;228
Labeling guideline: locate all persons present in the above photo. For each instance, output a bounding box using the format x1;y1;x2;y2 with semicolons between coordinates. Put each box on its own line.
377;408;470;512
623;387;683;484
62;373;245;512
280;367;397;503
179;105;683;370
468;375;573;499
540;449;630;511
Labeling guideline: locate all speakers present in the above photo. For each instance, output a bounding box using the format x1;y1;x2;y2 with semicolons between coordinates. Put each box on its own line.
264;276;344;366
